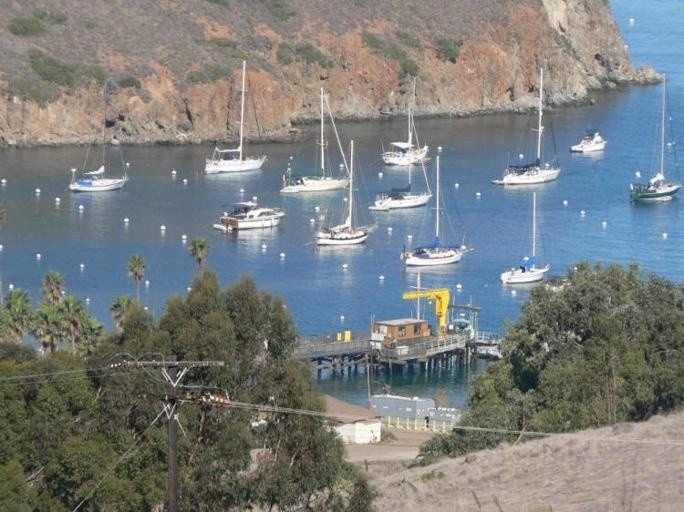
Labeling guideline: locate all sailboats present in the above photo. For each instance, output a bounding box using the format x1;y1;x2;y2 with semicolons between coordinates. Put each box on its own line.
631;74;681;204
382;78;427;165
491;68;563;185
400;155;462;267
203;60;268;174
316;141;368;247
69;84;129;192
279;88;351;194
501;193;551;284
368;132;433;210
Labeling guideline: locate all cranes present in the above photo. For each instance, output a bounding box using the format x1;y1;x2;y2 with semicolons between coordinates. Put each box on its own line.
403;288;450;333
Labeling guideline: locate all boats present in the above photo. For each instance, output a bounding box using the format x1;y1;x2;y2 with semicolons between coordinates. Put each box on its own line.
571;129;607;153
213;202;285;231
477;333;503;358
